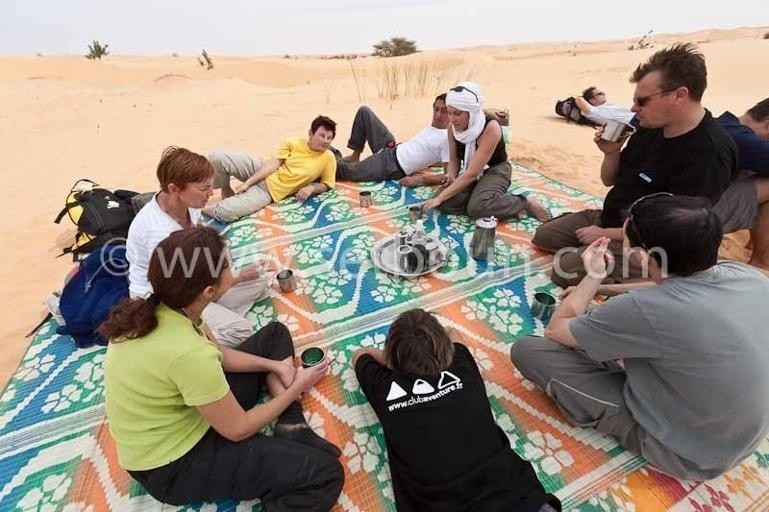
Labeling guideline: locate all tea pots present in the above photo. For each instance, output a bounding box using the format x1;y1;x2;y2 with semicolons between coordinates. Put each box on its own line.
469;216;498;263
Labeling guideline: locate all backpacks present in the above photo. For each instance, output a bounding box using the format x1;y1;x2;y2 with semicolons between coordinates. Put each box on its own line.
555;97;602;129
54;179;135;262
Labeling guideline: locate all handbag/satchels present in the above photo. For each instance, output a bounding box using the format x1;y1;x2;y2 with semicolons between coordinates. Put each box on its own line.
57;244;130;349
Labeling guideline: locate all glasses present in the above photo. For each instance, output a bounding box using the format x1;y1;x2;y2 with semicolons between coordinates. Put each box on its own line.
627;192;674;257
450;86;478;102
595;92;605;95
633;86;676;107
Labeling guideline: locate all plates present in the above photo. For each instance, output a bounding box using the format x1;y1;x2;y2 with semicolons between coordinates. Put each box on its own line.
370;233;450;278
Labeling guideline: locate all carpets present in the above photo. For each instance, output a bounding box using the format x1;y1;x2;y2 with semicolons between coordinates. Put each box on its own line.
0;162;769;512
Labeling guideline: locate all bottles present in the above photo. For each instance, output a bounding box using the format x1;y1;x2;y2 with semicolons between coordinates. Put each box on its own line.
411;220;427;272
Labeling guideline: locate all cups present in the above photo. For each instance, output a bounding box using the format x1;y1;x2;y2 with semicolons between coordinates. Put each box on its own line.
300;347;325;369
276;269;297;293
530;292;556;321
359;191;371;208
408;207;422;224
600;119;637;143
394;230;439;274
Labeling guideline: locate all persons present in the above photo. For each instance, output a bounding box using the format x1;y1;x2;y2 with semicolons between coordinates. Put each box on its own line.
511;196;769;481
95;227;345;512
201;115;337;224
418;81;550;223
531;41;738;288
336;93;450;188
352;307;563;511
710;96;769;271
573;86;639;128
126;145;265;349
